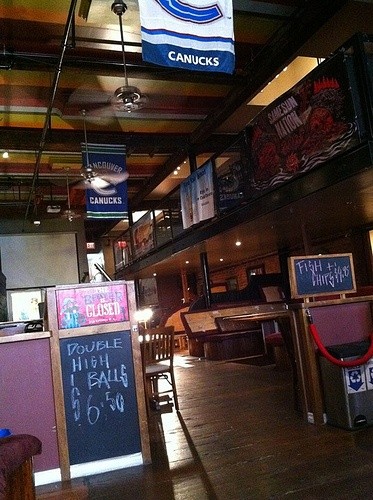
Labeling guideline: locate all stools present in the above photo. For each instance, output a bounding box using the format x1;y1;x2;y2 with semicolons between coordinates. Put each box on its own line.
146;345;173;412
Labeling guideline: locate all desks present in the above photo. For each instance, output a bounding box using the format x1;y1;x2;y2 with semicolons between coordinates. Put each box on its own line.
223;309;296;373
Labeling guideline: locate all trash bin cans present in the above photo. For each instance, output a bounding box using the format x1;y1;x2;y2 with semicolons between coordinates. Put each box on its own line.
317;339;373;430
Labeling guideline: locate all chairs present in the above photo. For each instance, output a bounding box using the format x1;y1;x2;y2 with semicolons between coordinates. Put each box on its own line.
137;325;180;413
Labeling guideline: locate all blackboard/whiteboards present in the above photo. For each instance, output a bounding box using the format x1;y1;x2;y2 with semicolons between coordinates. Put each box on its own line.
288;252;358;299
45;321;150;468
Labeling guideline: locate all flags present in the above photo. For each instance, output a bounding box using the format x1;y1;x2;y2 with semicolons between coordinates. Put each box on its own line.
81;143;128;219
138;0;234;74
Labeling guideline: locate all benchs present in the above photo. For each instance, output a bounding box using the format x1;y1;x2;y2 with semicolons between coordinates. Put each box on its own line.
179;301;295;361
